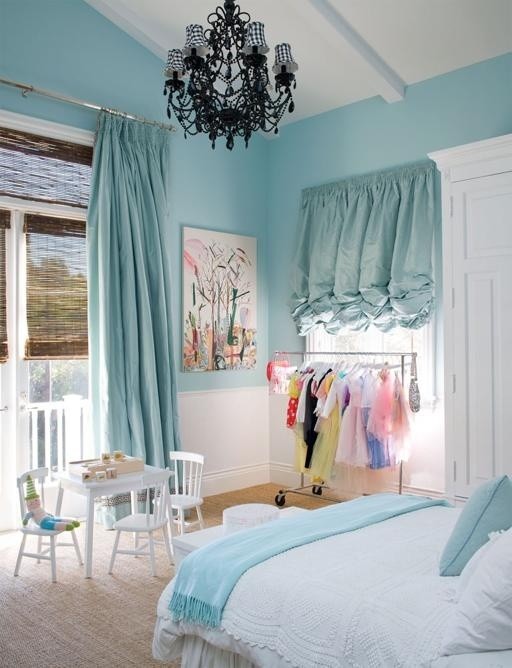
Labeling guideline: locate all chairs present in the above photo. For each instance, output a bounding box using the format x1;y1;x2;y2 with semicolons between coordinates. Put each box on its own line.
108;450;207;577
11;463;83;587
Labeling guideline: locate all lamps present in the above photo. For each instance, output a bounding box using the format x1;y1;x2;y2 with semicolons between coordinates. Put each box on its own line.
159;0;304;152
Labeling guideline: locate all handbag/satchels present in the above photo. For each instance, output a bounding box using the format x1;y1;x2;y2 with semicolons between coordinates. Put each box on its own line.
408;376;421;414
266;360;297;395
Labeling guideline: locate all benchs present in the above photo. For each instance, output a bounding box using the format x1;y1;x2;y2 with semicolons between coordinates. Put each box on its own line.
166;504;310;565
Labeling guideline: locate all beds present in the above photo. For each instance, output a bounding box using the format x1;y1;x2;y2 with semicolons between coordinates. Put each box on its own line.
156;478;511;667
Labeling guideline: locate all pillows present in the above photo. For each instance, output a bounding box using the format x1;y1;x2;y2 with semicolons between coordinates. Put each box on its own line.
437;476;509;654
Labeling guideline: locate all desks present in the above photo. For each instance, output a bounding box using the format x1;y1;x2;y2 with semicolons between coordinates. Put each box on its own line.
50;465;175;578
